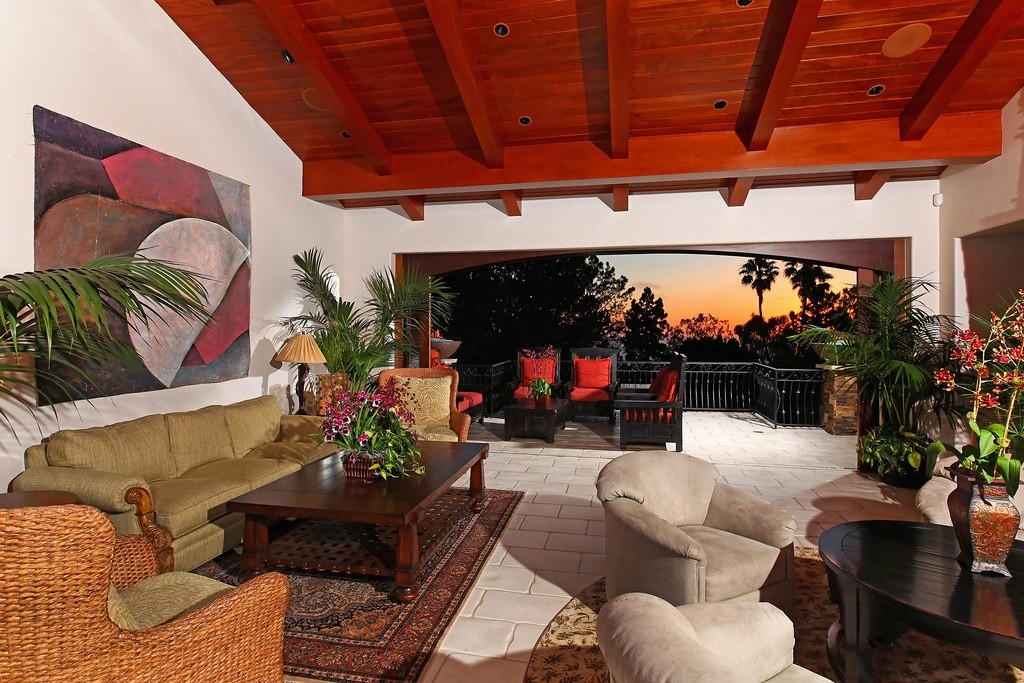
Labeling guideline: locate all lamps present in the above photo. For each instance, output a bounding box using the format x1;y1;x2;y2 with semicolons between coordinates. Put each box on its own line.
274;331;327;415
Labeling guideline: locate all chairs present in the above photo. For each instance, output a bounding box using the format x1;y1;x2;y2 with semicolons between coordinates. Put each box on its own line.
1;485;288;683
614;352;688;452
563;347;620;422
430;350;486;427
513;348;561;399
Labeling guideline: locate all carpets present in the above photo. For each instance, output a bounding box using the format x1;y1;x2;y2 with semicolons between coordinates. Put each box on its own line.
524;546;1024;683
185;487;524;683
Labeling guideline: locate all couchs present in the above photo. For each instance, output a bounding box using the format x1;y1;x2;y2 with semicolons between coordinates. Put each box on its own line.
593;450;797;616
592;590;835;683
378;365;470;444
6;394;346;572
915;406;1024;542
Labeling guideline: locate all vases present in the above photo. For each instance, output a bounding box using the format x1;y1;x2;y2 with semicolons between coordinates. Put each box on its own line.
533;394;547;406
942;466;1023;577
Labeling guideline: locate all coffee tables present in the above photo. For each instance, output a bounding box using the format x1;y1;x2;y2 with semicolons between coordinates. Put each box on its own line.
818;520;1024;683
225;441;491;602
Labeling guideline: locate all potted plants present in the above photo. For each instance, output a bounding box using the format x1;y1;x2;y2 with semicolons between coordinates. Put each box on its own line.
781;259;968;488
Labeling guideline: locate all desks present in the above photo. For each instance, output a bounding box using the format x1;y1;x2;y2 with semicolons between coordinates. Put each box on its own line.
498;399;569;443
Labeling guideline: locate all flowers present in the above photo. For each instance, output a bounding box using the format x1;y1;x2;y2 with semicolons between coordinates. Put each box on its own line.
933;290;1024;500
522;345;556;399
305;388;426;481
